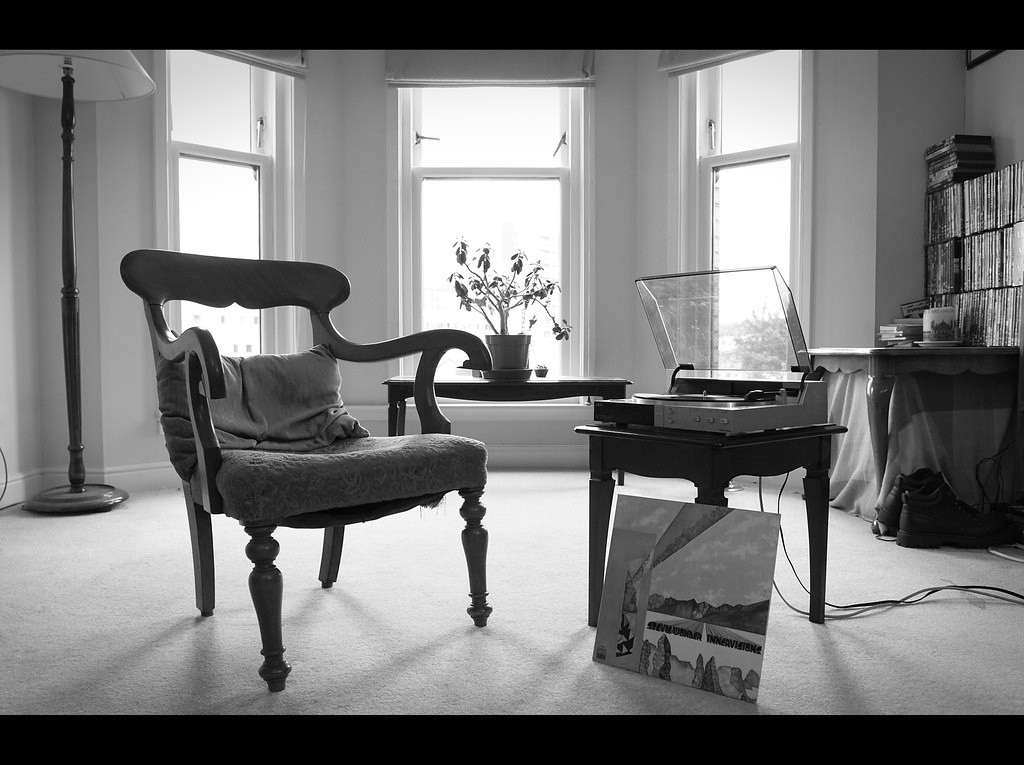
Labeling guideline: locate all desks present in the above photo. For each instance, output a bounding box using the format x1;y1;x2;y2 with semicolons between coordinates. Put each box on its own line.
382;372;634;487
795;341;1016;538
575;416;849;632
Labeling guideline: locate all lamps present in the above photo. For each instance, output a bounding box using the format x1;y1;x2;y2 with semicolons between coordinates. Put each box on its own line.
0;51;157;517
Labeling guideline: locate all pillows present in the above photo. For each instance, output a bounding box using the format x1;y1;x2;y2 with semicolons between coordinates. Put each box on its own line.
157;331;370;482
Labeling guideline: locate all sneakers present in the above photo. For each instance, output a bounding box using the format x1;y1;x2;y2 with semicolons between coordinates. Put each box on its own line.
897;477;1005;548
877;468;942;529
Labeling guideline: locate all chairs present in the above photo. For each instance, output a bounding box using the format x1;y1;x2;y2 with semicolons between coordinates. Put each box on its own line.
120;250;495;693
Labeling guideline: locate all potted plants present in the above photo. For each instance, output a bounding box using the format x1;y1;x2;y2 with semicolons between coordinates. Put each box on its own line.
535;361;549;377
448;236;574;371
454;361;476;376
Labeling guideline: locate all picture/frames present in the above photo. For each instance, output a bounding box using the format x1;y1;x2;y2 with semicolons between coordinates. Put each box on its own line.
964;50;1003;71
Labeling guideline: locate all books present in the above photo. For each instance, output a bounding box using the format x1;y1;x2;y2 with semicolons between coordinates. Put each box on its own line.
876;133;1023;349
